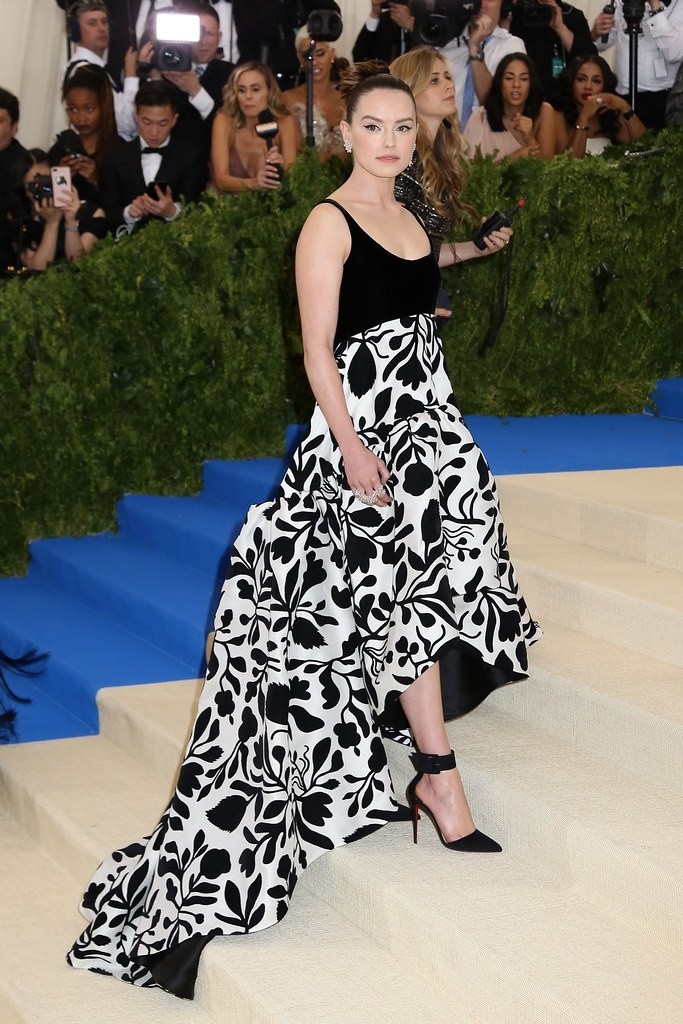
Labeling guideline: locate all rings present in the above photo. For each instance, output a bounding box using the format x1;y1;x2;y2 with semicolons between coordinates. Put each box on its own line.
528;148;532;156
483;25;488;29
504;240;509;245
351;484;387;505
596;97;602;104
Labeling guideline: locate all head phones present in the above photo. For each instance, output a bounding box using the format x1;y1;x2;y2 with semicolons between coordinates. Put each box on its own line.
66;3;81;43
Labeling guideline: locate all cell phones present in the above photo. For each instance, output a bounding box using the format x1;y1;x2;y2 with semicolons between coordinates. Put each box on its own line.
52;166;72;206
64;145;81;159
148;182;168;201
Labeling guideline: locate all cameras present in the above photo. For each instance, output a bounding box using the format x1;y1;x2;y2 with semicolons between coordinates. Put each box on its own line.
153;12;201;71
413;10;454;48
29;173;53;205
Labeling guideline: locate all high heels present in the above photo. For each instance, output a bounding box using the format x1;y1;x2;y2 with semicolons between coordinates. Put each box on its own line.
406;750;503;855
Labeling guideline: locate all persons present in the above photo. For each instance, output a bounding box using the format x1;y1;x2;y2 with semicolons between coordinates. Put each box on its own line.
352;0;683;166
389;43;485;323
44;0;343;152
5;148;105;271
292;64;516;855
0;84;35;282
47;68;133;221
276;20;354;173
99;80;209;242
210;60;301;195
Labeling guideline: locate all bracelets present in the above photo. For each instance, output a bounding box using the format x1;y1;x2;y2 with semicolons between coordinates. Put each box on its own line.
574;125;590;132
406;15;414;31
647;7;664;16
466;50;484;64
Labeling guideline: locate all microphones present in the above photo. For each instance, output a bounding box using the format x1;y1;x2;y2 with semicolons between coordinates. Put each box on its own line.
256;110;279;174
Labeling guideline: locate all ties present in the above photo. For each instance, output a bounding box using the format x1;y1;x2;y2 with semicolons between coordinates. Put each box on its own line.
460;36;495;135
192;66;205;80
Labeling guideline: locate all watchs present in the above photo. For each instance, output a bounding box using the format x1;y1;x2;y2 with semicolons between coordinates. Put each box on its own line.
63;220;81;234
621;107;636;122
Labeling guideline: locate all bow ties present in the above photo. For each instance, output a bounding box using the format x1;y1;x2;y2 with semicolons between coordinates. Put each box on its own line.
138;145;169;157
